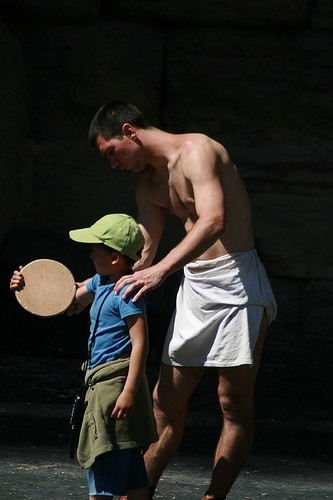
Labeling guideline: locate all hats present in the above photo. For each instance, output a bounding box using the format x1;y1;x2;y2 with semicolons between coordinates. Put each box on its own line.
69;213;145;262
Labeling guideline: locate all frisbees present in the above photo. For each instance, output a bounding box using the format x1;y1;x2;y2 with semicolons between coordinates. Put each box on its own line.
15;259;76;317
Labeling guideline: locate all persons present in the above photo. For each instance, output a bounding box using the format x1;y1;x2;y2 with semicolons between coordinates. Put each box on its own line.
62;101;277;500
9;214;159;500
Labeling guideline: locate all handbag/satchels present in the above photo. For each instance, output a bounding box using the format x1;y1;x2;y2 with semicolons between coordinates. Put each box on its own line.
70;394;87;434
